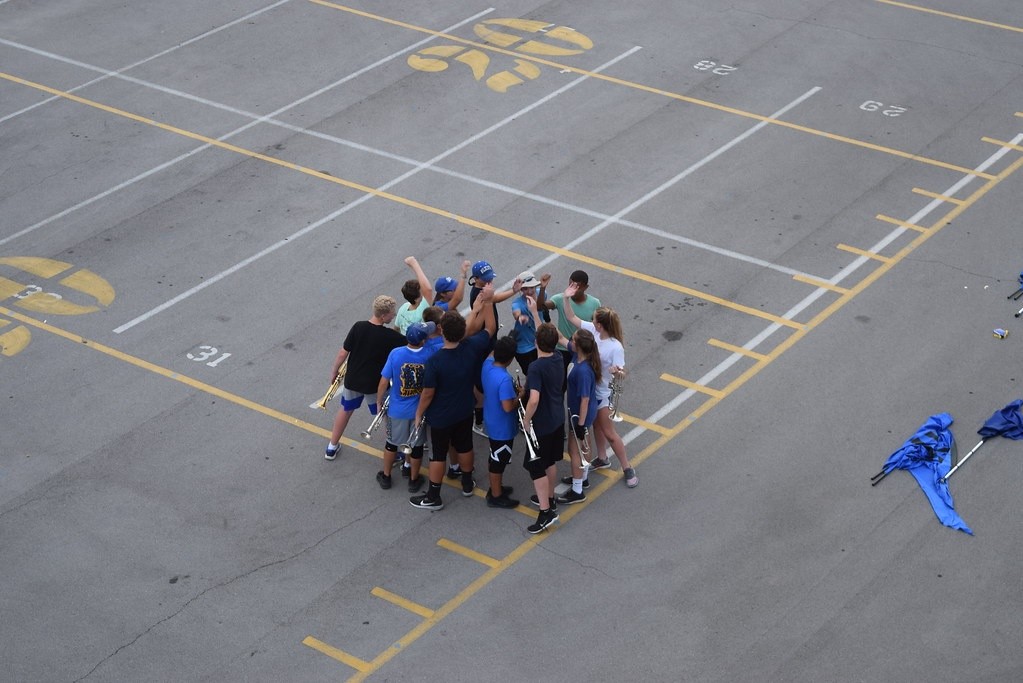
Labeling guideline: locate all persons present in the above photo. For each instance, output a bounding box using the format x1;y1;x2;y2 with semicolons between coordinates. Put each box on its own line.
432;260;471;312
469;260;524;437
537;271;601;366
518;295;567;533
393;256;433;336
324;295;408;460
512;272;551;376
375;283;497;510
556;328;602;505
482;337;524;509
563;282;638;487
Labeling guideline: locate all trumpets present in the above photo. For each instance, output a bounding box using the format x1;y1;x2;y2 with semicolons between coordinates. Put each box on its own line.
316;361;349;411
516;397;543;465
398;414;426;455
607;368;626;424
566;406;600;472
360;394;391;442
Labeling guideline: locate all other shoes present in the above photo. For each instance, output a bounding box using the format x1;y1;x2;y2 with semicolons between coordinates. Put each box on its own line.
402;465;412;476
408;474;426;493
377;472;393;489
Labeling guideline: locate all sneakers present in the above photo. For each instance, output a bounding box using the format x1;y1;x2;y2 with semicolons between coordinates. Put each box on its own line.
325;442;342;460
447;464;475;480
409;490;443;510
561;476;591;488
392;451;405;467
556;488;586;505
530;494;558;511
527;508;559;533
472;423;489;437
462;480;476;496
485;485;513;499
623;465;638;487
588;456;611;471
486;493;520;509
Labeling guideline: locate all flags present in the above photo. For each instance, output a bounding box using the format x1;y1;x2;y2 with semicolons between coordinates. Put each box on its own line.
884;412;973;535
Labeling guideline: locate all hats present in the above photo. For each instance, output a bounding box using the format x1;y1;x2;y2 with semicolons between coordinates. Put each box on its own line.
435;275;458;293
518;271;542;289
472;261;497;282
406;321;436;344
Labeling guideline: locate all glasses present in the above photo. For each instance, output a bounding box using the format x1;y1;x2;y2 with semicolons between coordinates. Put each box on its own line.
522;276;536;284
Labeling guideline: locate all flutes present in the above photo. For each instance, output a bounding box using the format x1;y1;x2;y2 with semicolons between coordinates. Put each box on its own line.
515;367;523;392
